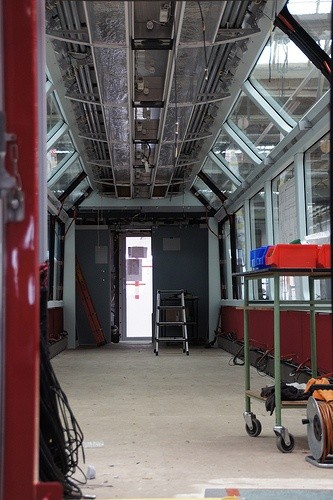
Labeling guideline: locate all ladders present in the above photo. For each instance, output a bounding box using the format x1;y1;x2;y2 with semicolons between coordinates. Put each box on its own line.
154;289;189;356
75;259;107;347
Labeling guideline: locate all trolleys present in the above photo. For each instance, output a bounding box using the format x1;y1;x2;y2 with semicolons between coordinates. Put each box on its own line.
231;266;333;455
